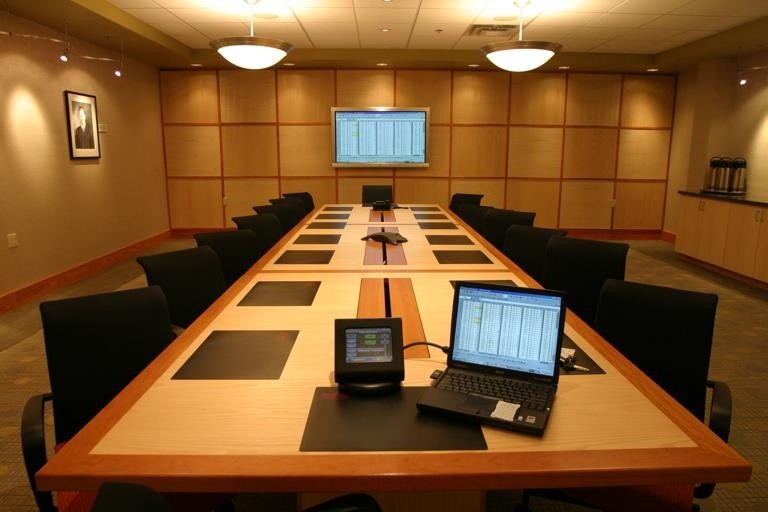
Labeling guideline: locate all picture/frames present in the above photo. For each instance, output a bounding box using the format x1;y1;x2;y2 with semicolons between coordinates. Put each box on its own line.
65;90;100;160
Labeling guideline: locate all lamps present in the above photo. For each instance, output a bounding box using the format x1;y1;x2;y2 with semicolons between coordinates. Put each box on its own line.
59;17;72;62
209;0;293;70
114;38;129;77
480;0;564;73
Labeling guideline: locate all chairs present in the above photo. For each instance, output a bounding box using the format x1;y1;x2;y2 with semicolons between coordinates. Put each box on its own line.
232;190;315;248
517;237;631;285
571;280;732;512
192;227;262;288
22;284;178;511
449;191;568;265
136;245;228;334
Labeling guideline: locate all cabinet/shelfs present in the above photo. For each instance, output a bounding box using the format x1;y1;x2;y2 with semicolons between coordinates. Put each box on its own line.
673;190;768;290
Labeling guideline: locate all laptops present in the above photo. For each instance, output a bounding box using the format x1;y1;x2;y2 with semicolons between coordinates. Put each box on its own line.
416;279;570;438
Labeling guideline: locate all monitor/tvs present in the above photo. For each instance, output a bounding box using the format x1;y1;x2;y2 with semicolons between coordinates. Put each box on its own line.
331;106;430;169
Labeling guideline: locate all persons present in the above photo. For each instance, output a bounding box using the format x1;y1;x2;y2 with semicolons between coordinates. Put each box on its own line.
75;106;94;148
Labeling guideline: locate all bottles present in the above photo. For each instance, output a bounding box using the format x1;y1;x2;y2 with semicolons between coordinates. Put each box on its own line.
706;156;746;192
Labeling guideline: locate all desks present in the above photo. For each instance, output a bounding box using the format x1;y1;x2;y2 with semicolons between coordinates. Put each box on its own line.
34;204;752;511
